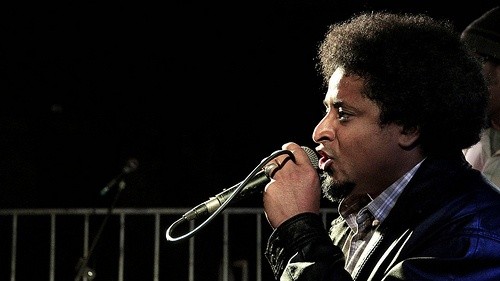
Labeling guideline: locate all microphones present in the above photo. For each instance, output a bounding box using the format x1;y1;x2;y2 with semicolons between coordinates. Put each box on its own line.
98;156;141;195
182;144;319;221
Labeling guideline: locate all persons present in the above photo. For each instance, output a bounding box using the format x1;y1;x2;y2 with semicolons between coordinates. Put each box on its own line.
261;13;500;280
461;7;500;189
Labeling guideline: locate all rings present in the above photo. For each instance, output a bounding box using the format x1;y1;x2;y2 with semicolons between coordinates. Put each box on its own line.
265;163;277;177
